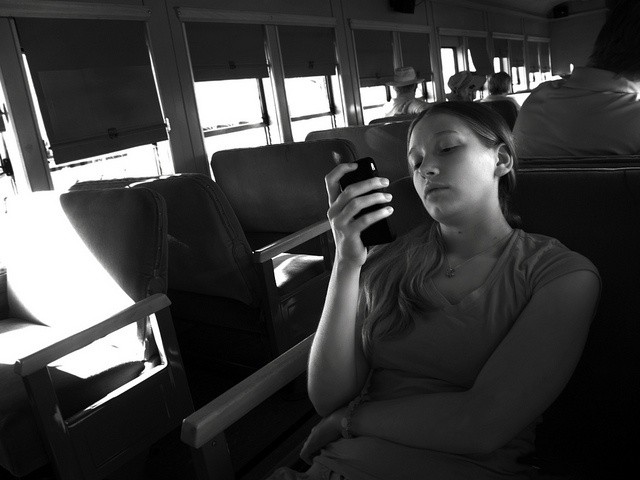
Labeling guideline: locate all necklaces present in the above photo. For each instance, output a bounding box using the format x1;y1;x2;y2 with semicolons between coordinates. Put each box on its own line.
442;230;515;279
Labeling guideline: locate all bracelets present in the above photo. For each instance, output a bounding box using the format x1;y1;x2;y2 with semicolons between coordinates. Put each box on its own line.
339;394;372;438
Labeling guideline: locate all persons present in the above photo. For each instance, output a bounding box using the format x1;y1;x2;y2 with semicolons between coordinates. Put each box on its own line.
445;71;483;108
299;100;602;479
512;2;639;156
478;72;521;116
379;66;432;116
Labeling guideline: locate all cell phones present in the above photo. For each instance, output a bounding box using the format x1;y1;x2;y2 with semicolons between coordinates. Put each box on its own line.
338;156;397;247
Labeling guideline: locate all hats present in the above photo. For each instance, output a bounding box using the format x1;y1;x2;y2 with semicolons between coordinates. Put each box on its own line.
386;66;425;87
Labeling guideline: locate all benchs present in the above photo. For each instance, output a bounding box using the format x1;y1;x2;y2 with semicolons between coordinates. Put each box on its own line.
303;120;412;185
181;167;639;480
481;100;518;132
211;139;432;238
1;189;195;480
68;173;332;359
